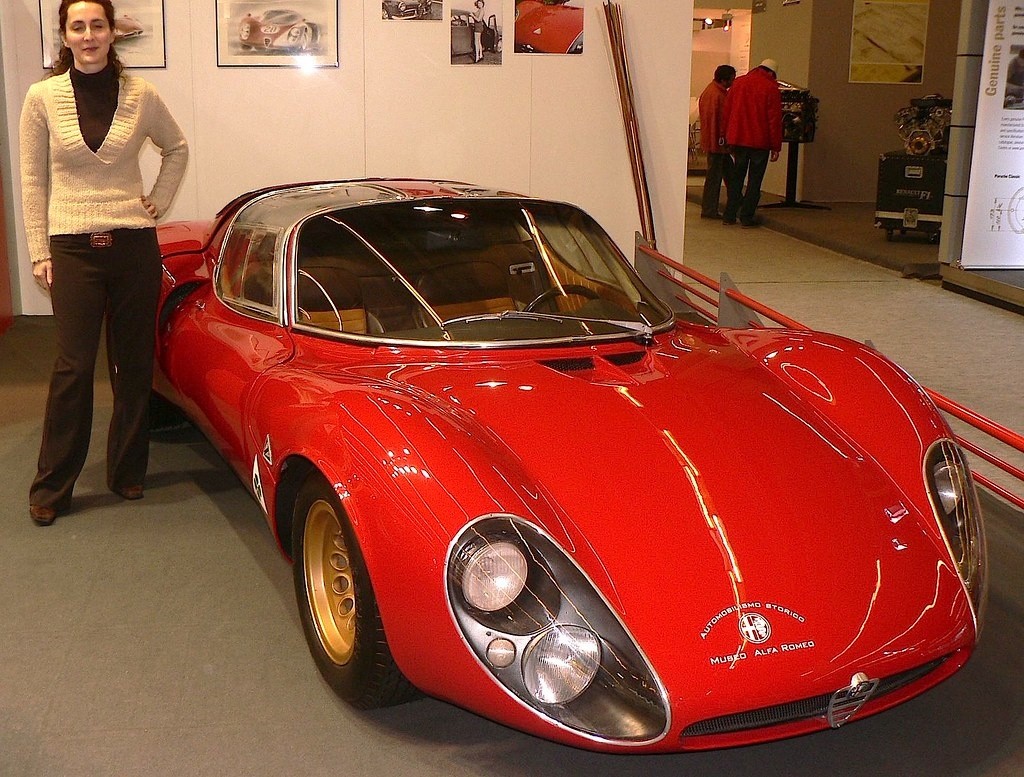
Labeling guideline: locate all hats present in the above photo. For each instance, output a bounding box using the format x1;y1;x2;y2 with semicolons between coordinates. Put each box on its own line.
760;58;779;80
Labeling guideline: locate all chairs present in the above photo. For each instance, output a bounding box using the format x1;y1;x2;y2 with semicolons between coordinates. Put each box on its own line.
244;267;388;336
412;260;534;328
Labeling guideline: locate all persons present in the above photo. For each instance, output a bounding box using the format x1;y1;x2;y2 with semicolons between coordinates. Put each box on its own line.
723;58;783;228
468;0;485;64
699;65;744;220
19;0;187;524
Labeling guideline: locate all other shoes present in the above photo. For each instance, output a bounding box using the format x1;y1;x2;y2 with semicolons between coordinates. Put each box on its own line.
30;502;56;523
741;217;761;228
118;482;142;499
723;216;737;225
701;211;723;219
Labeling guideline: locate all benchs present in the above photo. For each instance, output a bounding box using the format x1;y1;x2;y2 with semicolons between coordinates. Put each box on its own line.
299;221;559;330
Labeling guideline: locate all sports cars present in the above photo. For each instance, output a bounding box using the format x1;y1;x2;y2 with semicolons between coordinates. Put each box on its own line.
515;0;583;55
239;9;327;55
107;176;991;757
110;16;147;43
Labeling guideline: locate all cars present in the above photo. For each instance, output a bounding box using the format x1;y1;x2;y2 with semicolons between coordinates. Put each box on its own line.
451;8;502;56
382;0;432;20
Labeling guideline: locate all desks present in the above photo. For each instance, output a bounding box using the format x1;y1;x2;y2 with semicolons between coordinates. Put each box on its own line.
875;147;948;243
